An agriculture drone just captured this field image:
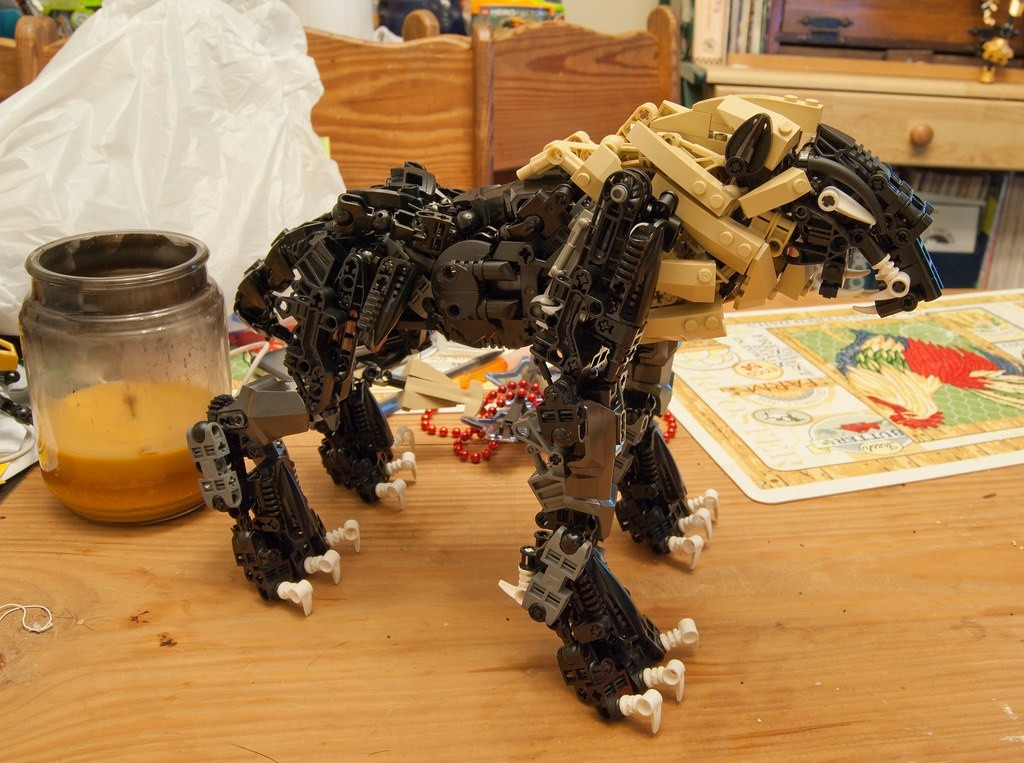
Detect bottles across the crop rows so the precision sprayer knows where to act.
[19,230,230,525]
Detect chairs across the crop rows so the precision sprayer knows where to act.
[402,3,683,179]
[0,14,61,103]
[225,22,493,191]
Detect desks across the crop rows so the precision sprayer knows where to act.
[699,54,1024,173]
[1,286,1024,762]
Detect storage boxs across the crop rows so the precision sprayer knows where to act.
[910,191,987,256]
